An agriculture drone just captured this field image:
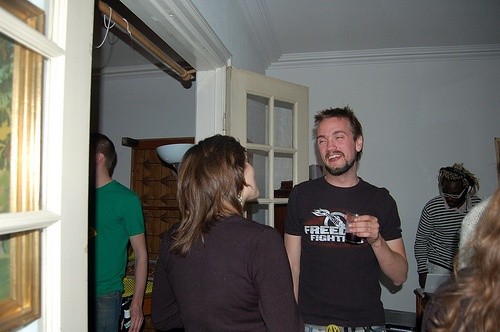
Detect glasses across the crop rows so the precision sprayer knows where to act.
[440,187,467,199]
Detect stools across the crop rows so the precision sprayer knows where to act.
[384,309,417,332]
[414,288,435,332]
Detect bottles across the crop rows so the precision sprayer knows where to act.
[122,307,132,332]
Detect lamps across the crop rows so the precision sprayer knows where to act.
[156,143,195,173]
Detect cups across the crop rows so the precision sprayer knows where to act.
[345,212,367,243]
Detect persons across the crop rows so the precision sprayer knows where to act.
[89,132,148,332]
[151,135,305,332]
[414,163,500,332]
[282,108,408,332]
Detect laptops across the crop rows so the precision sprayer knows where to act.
[421,274,451,293]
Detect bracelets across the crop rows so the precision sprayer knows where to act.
[369,234,380,243]
